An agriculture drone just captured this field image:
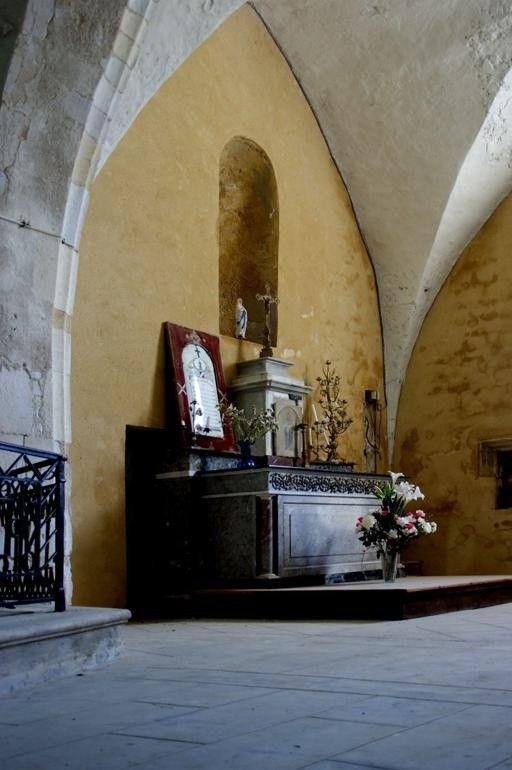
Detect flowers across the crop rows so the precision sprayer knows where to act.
[214,401,280,442]
[354,470,438,558]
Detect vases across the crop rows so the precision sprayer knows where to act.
[380,540,399,582]
[236,439,255,469]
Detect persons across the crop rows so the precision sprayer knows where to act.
[234,296,248,339]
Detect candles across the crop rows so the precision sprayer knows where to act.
[312,403,318,421]
[323,432,330,445]
[308,427,313,446]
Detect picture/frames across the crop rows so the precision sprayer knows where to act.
[164,321,235,452]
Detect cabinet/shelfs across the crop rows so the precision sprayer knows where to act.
[155,466,413,586]
